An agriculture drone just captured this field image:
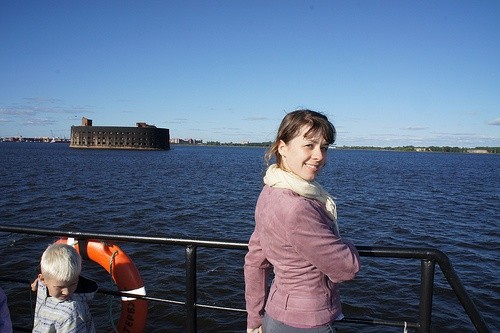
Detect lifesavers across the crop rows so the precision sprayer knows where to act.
[48,237,148,333]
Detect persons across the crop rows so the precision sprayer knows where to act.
[242,108,361,333]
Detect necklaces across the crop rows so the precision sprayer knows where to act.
[32,243,100,333]
